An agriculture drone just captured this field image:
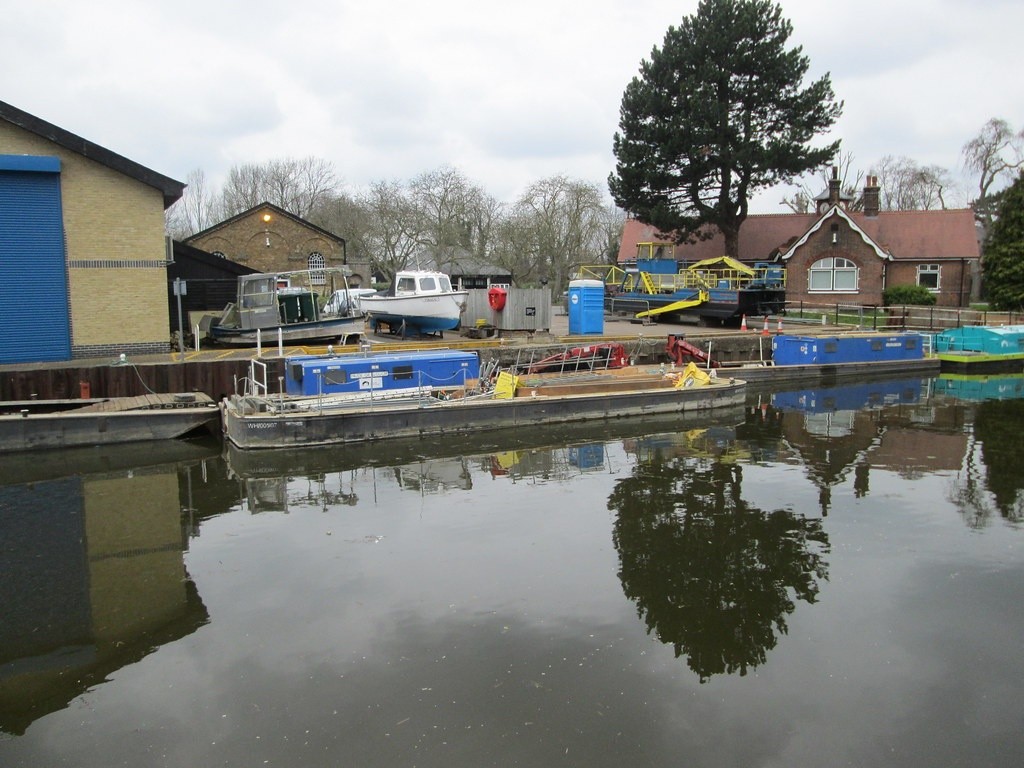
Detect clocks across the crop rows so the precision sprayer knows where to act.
[840,201,847,212]
[819,202,830,216]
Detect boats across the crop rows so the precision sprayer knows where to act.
[929,323,1024,371]
[529,327,941,385]
[1,388,216,451]
[206,267,366,346]
[357,269,470,337]
[219,334,748,448]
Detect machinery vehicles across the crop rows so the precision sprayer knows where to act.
[601,239,788,329]
